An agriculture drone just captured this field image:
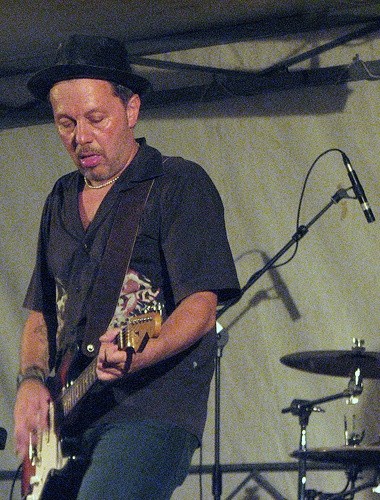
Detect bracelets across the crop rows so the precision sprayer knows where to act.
[17,365,46,388]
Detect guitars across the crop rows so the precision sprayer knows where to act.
[21,302,165,500]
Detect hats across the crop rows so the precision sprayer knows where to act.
[26,34,152,110]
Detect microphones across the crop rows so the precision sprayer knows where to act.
[341,151,376,225]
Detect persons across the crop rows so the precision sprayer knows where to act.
[12,33,243,500]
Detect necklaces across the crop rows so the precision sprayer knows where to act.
[83,176,120,190]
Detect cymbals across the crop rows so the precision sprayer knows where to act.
[288,446,380,464]
[281,351,380,378]
